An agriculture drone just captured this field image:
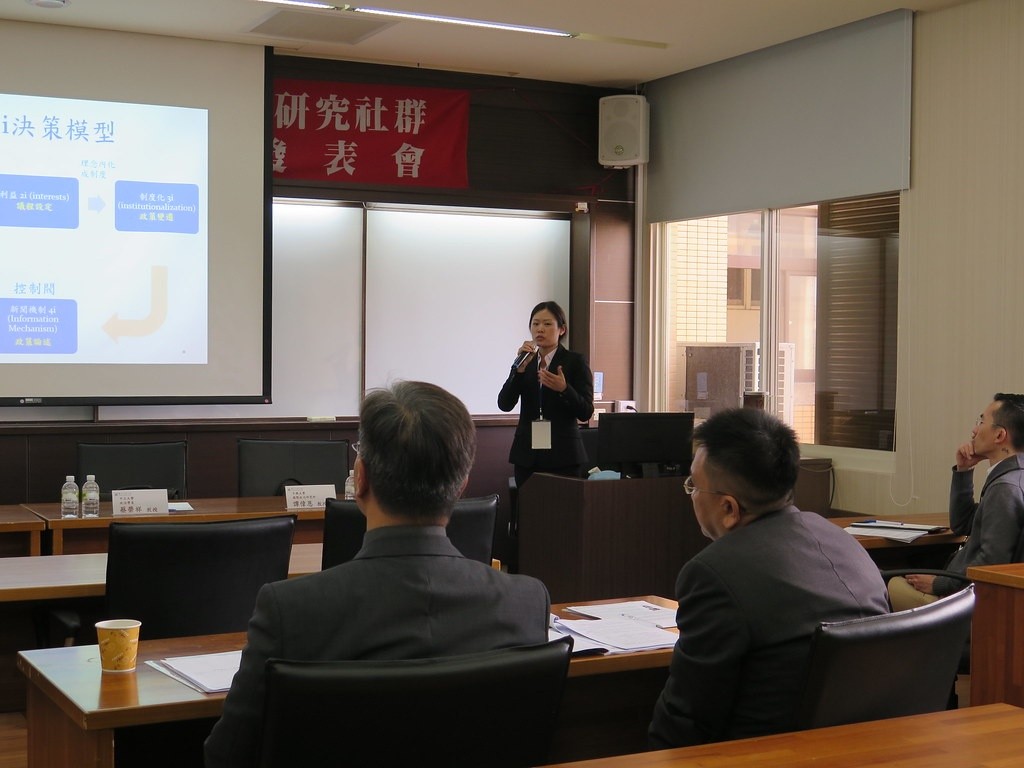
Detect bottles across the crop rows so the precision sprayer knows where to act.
[61,476,79,518]
[344,470,356,500]
[82,475,99,518]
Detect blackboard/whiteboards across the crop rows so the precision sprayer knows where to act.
[93,198,592,427]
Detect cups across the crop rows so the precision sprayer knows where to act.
[95,620,141,672]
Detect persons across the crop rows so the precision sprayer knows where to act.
[497,302,595,491]
[644,406,891,751]
[887,392,1024,614]
[201,379,549,768]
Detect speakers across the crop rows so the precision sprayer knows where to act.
[598,95,650,169]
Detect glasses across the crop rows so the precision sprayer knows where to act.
[682,475,742,511]
[351,441,361,457]
[976,417,1007,435]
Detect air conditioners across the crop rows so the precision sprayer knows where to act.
[684,343,795,431]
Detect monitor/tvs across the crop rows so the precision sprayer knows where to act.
[596,412,694,480]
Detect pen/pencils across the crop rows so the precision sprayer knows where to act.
[621,613,664,630]
[875,520,904,525]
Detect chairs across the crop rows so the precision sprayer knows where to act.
[237,437,349,498]
[797,581,977,729]
[74,441,189,500]
[322,492,500,573]
[36,510,300,648]
[261,637,575,767]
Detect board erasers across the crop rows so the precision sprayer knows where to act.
[307,416,336,422]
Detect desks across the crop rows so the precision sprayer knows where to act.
[1,492,1023,767]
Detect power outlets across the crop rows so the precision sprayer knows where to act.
[614,400,637,412]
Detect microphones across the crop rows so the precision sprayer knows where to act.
[512,339,537,370]
